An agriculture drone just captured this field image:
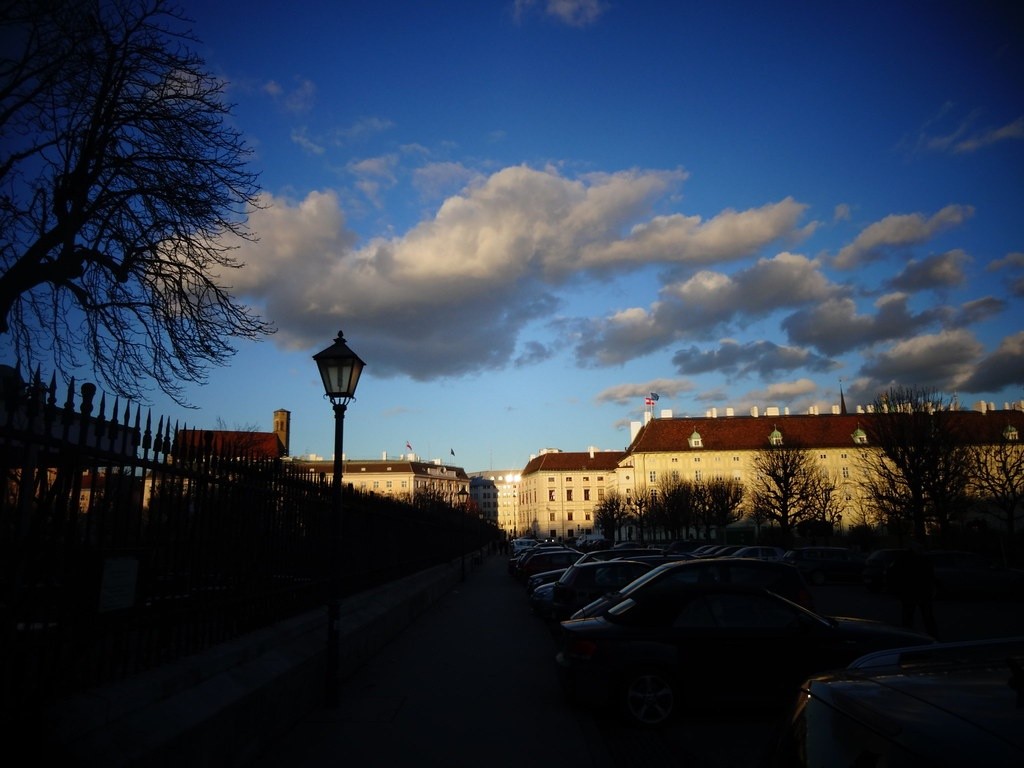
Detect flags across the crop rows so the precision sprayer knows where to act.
[645,397,655,406]
[407,441,412,451]
[451,448,455,456]
[650,392,660,401]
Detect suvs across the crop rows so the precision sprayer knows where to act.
[863,548,944,594]
[781,546,867,587]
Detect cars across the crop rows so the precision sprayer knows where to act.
[552,583,942,733]
[761,635,1024,768]
[690,543,786,558]
[561,557,818,621]
[508,536,694,622]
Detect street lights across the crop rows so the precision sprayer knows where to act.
[458,486,468,583]
[478,510,484,563]
[486,516,497,556]
[313,331,366,711]
